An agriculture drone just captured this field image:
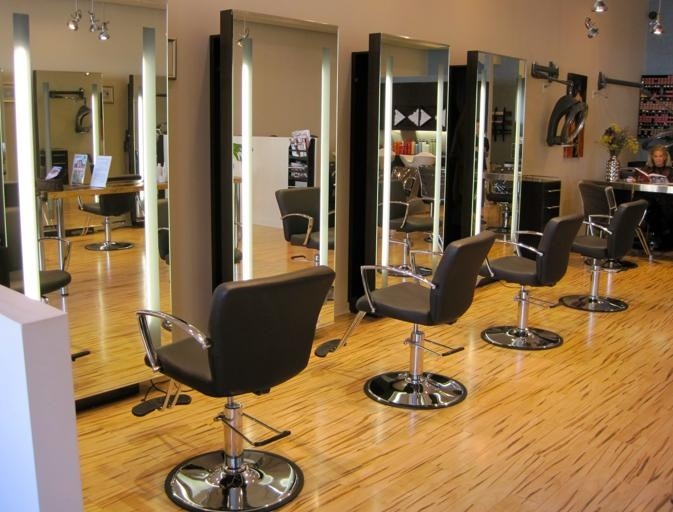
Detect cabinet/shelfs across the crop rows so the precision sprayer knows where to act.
[520,174,561,262]
[288,138,314,188]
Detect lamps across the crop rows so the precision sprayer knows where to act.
[582,16,599,39]
[646,0,665,35]
[590,0,608,12]
[66,0,110,41]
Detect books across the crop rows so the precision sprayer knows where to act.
[290,130,311,180]
[635,167,669,183]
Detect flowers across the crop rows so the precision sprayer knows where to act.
[593,121,637,157]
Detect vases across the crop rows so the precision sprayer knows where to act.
[603,155,620,182]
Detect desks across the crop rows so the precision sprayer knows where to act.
[43,182,168,298]
[577,177,672,261]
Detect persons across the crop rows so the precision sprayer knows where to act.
[626,144,673,183]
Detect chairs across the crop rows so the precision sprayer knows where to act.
[76,191,138,252]
[269,184,338,303]
[5,205,89,361]
[557,199,646,312]
[122,267,338,510]
[417,164,445,243]
[484,173,513,234]
[475,210,590,350]
[575,181,653,271]
[313,229,496,409]
[377,178,443,277]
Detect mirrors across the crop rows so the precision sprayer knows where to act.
[31,69,106,190]
[209,8,338,332]
[132,76,166,223]
[0,0,173,409]
[468,51,527,285]
[357,32,449,319]
